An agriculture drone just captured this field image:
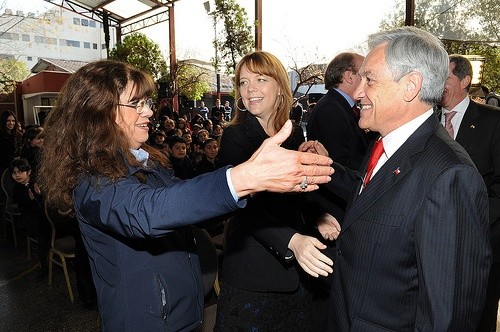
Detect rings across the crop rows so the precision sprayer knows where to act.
[300,176,307,189]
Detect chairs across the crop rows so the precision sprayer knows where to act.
[44,195,77,301]
[1,167,21,246]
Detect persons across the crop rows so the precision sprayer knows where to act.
[435,54,500,332]
[309,52,375,178]
[41,60,335,332]
[290,98,303,126]
[1,98,234,308]
[214,52,342,332]
[303,99,317,118]
[479,85,500,107]
[331,25,490,332]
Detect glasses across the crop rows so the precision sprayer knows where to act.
[118,99,152,115]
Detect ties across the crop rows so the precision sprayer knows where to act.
[363,138,384,189]
[443,111,457,140]
